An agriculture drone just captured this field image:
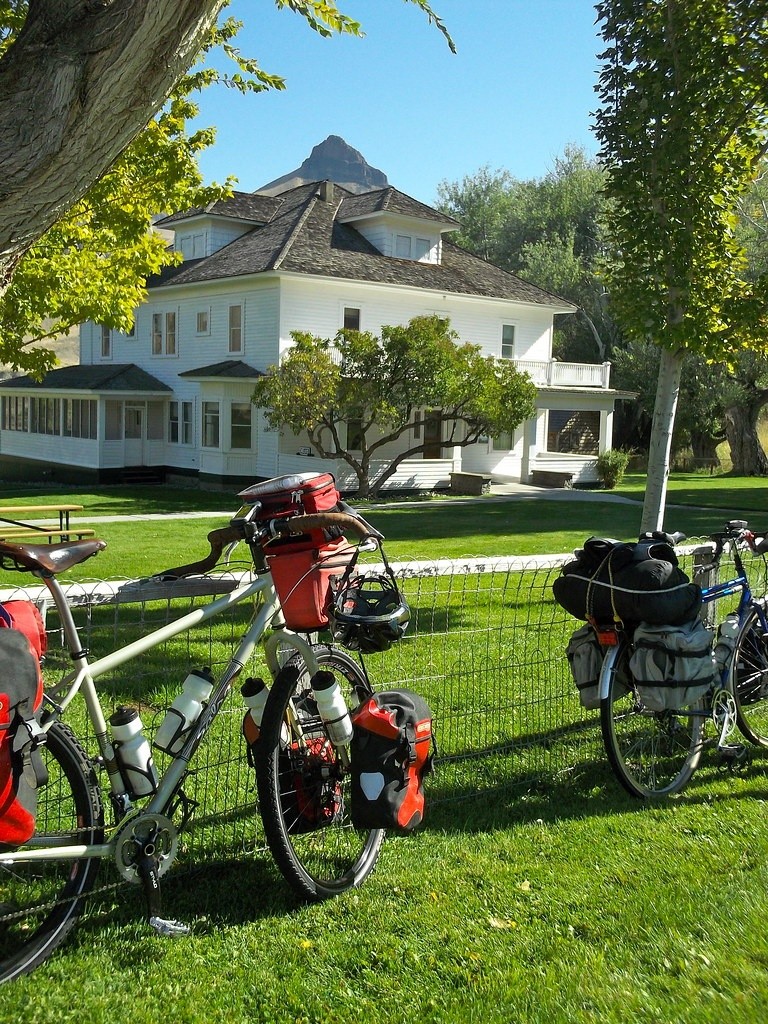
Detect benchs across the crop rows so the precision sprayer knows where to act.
[0,528,96,552]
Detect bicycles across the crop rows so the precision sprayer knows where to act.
[1,495,386,984]
[596,518,766,798]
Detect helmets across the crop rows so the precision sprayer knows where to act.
[324,573,412,655]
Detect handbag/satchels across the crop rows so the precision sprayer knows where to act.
[242,708,343,835]
[236,471,348,555]
[0,599,47,854]
[349,688,438,831]
[629,614,716,713]
[266,535,359,632]
[564,623,636,710]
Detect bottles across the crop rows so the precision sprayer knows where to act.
[308,671,355,744]
[713,611,740,670]
[108,706,158,796]
[154,663,215,755]
[241,677,292,751]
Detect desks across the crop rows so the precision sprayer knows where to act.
[0,503,84,544]
